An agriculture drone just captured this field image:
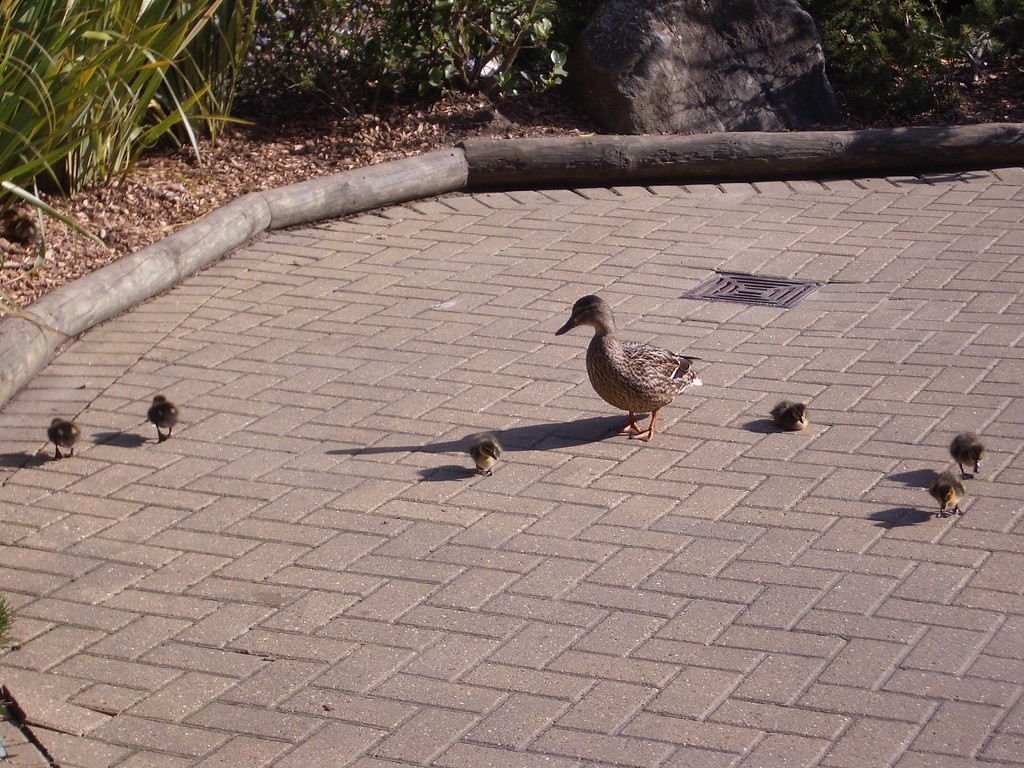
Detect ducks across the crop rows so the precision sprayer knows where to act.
[47,417,81,461]
[768,400,809,432]
[929,470,966,518]
[470,433,501,477]
[949,432,985,481]
[555,295,705,443]
[146,394,179,443]
